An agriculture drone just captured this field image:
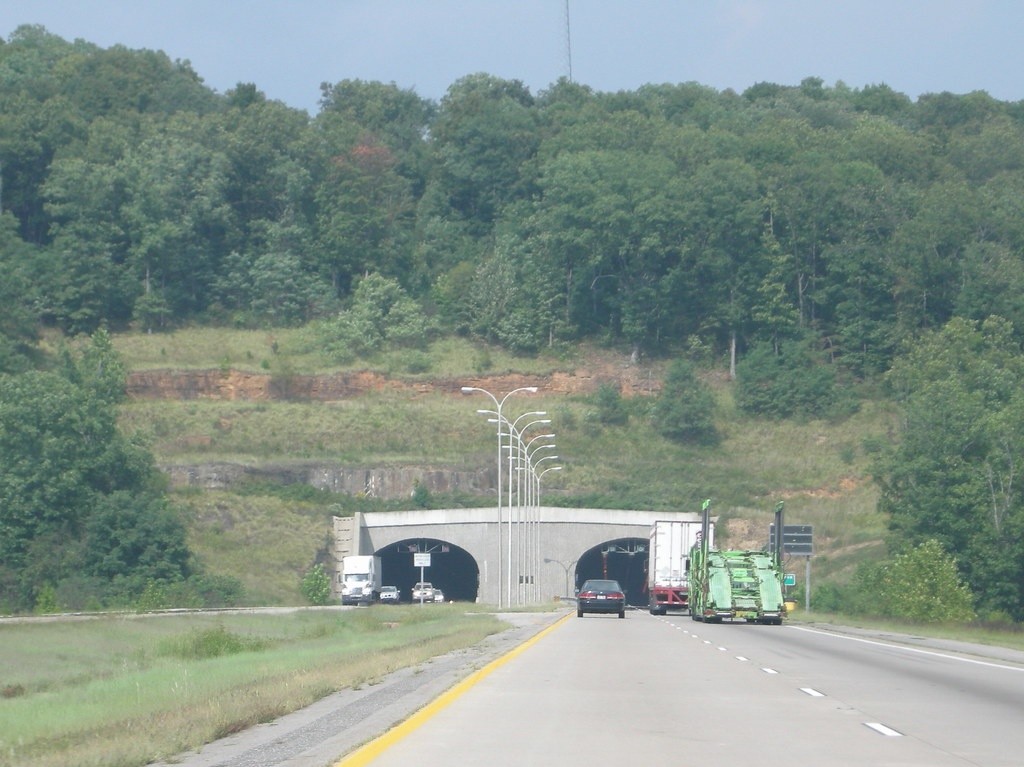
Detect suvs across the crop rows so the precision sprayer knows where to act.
[411,581,436,603]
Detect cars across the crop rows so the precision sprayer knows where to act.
[379,585,402,605]
[573,579,628,619]
[434,589,445,602]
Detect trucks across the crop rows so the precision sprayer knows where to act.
[643,520,716,616]
[337,556,383,606]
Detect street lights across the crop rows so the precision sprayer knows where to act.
[515,465,563,601]
[496,432,556,606]
[476,409,546,608]
[506,455,560,603]
[487,419,551,606]
[462,386,538,610]
[503,444,555,604]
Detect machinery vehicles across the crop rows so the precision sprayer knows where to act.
[683,497,786,625]
